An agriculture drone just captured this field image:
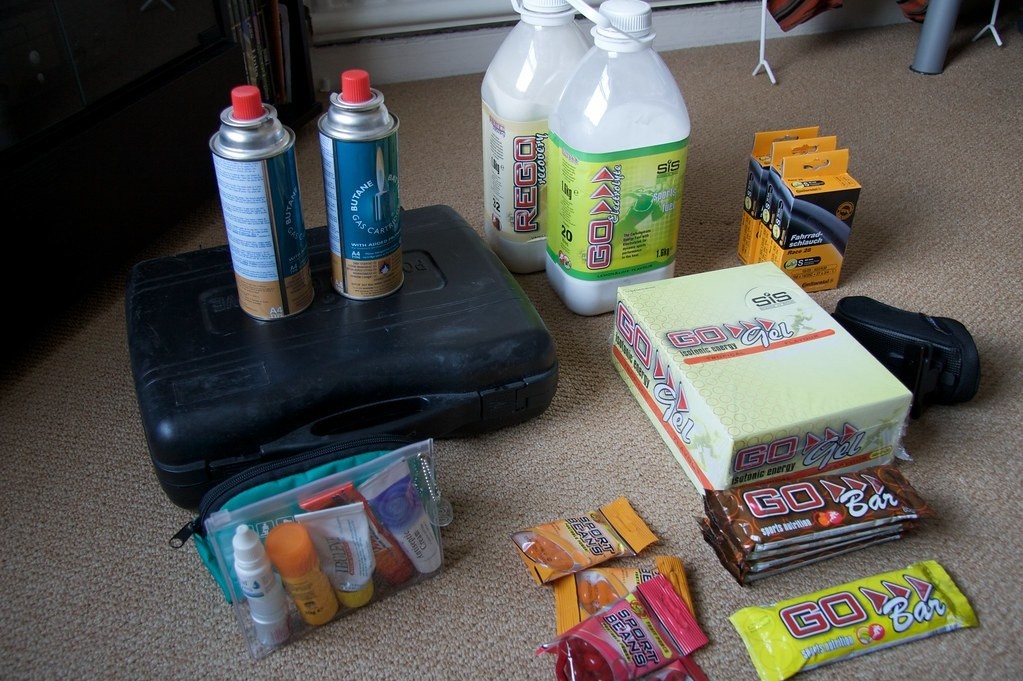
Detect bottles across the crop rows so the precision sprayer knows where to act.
[317,69,404,301]
[231,524,275,599]
[248,572,292,646]
[209,85,315,321]
[265,521,338,626]
[546,0,690,316]
[481,0,594,275]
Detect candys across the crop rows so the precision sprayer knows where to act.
[510,462,981,681]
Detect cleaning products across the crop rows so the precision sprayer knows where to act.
[480,0,692,318]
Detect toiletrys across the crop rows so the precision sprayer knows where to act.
[204,438,454,663]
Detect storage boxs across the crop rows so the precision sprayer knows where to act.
[123,201,559,512]
[736,126,862,288]
[611,259,913,498]
